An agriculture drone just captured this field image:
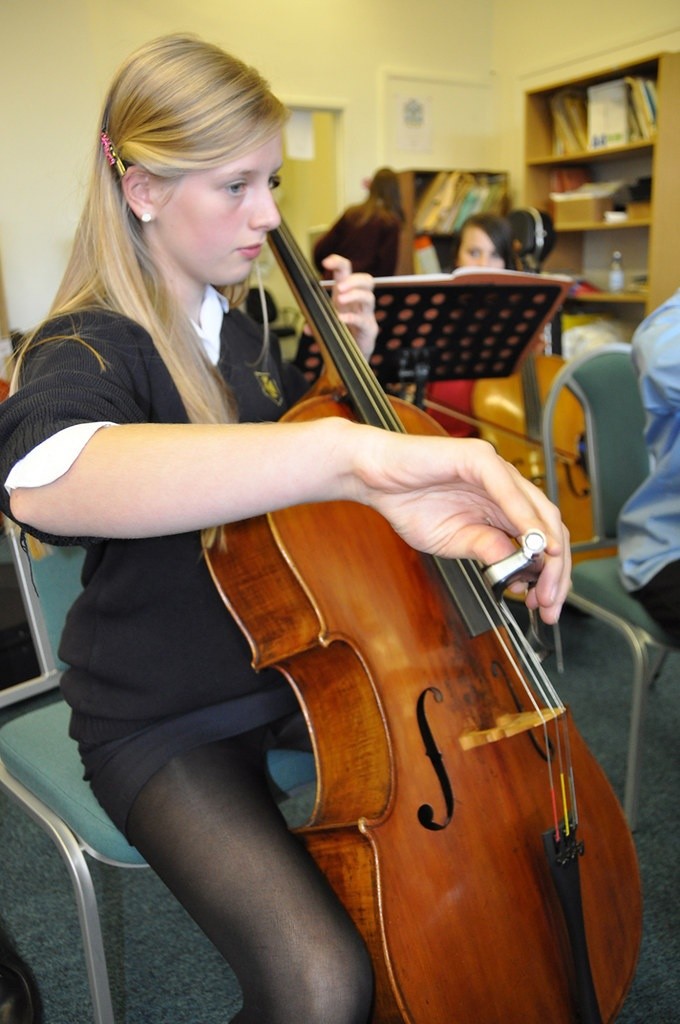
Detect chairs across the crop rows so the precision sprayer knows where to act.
[247,287,297,337]
[1,517,317,1023]
[544,350,680,834]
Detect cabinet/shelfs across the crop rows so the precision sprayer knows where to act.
[393,170,511,276]
[524,51,680,318]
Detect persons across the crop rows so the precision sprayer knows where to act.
[1,35,571,1023]
[617,289,680,644]
[311,167,513,278]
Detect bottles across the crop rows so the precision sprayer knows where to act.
[609,251,626,295]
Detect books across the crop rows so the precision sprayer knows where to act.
[547,73,658,155]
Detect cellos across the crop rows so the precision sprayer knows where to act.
[201,201,644,1023]
[470,237,617,601]
